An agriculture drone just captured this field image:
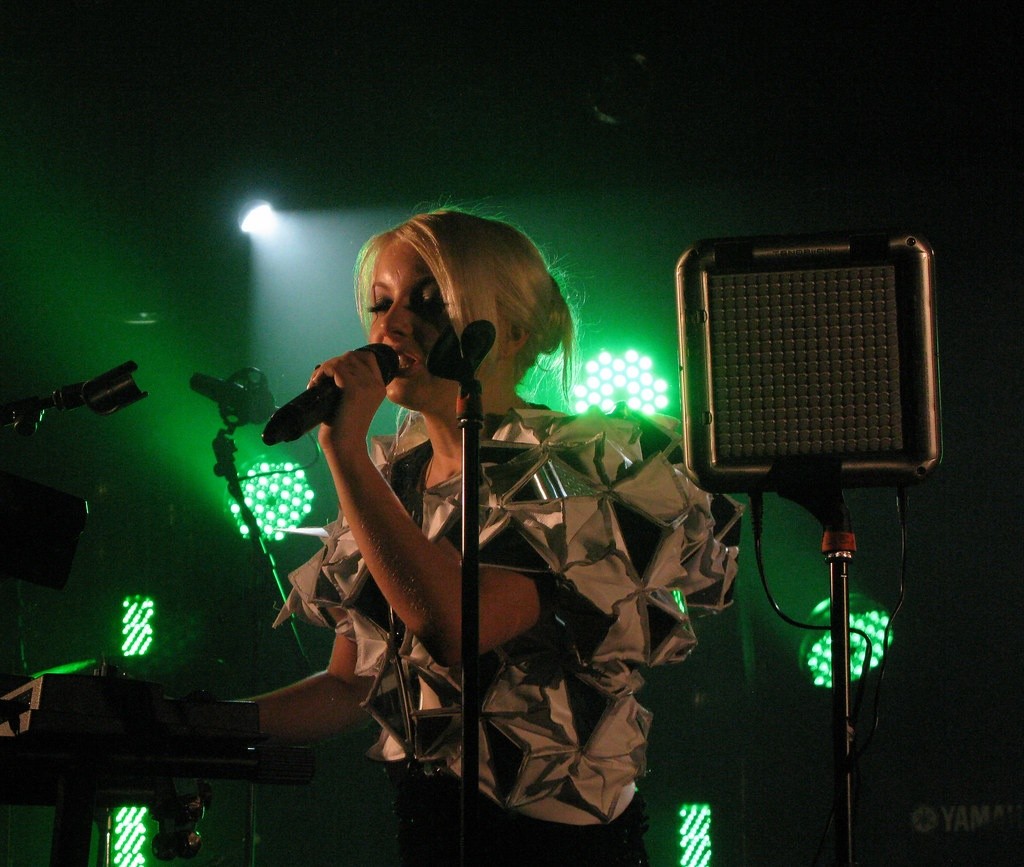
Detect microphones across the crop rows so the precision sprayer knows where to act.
[261,343,399,447]
[186,373,283,422]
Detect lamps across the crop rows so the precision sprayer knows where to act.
[798,586,896,689]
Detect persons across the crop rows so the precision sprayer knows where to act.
[134,206,735,867]
